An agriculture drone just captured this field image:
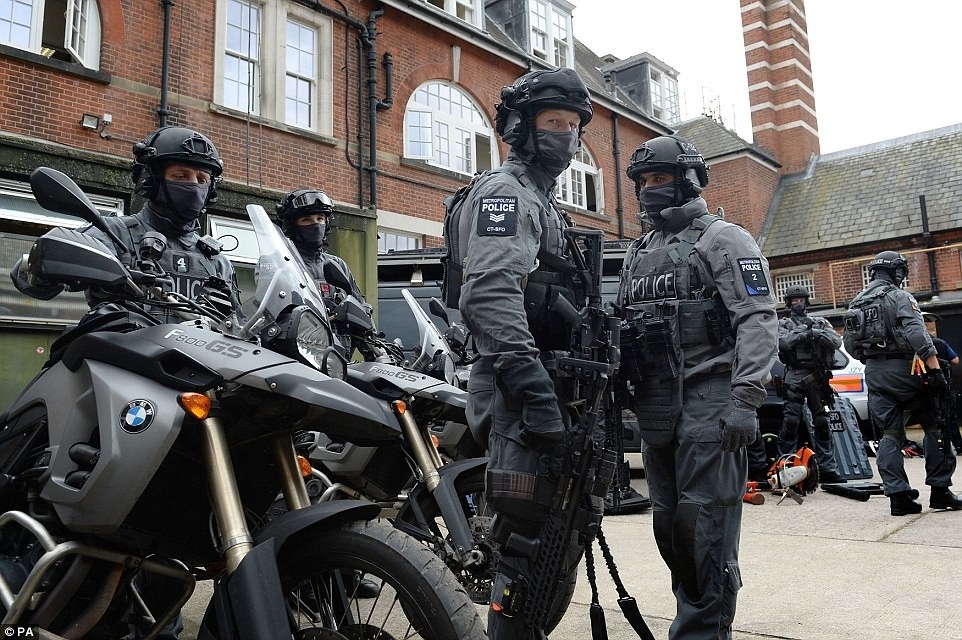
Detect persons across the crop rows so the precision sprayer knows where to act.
[779,285,845,481]
[10,128,243,320]
[453,67,595,640]
[273,188,372,362]
[846,252,962,515]
[615,137,778,640]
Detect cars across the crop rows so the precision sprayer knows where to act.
[757,336,874,441]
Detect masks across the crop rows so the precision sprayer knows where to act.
[295,223,326,248]
[524,126,578,163]
[638,181,684,216]
[157,179,210,217]
[793,303,804,312]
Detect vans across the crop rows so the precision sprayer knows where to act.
[377,239,644,454]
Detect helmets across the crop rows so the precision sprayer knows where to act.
[280,191,331,221]
[627,137,710,187]
[502,67,593,148]
[785,286,808,296]
[868,251,909,278]
[135,126,222,196]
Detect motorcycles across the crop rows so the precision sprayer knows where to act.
[0,165,496,640]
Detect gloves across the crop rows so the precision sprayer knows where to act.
[929,371,945,399]
[718,398,758,453]
[522,400,566,440]
[797,330,813,343]
[803,318,815,328]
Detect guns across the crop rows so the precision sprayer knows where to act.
[797,341,838,412]
[485,357,611,640]
[770,374,784,398]
[922,359,962,456]
[547,227,622,425]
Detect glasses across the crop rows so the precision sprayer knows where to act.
[292,192,332,208]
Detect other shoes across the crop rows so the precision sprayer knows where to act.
[818,471,847,485]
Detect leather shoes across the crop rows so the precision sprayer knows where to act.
[929,486,962,508]
[891,491,922,516]
